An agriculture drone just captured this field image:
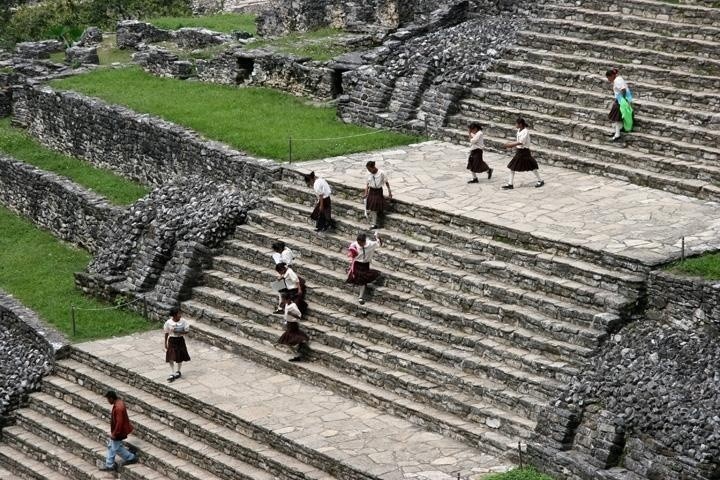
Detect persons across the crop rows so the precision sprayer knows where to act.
[276,295,309,362]
[606,69,634,142]
[161,308,193,381]
[466,121,494,184]
[99,390,137,472]
[303,170,338,232]
[274,262,309,312]
[501,117,545,190]
[269,240,295,270]
[342,232,383,304]
[359,159,393,229]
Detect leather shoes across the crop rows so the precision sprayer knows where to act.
[288,357,301,361]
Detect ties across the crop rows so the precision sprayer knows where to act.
[362,247,366,260]
[373,176,378,188]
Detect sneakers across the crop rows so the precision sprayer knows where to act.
[467,177,480,183]
[534,179,545,187]
[612,135,621,142]
[99,456,137,472]
[487,168,493,179]
[358,297,364,305]
[166,375,175,381]
[500,183,514,190]
[174,371,182,379]
